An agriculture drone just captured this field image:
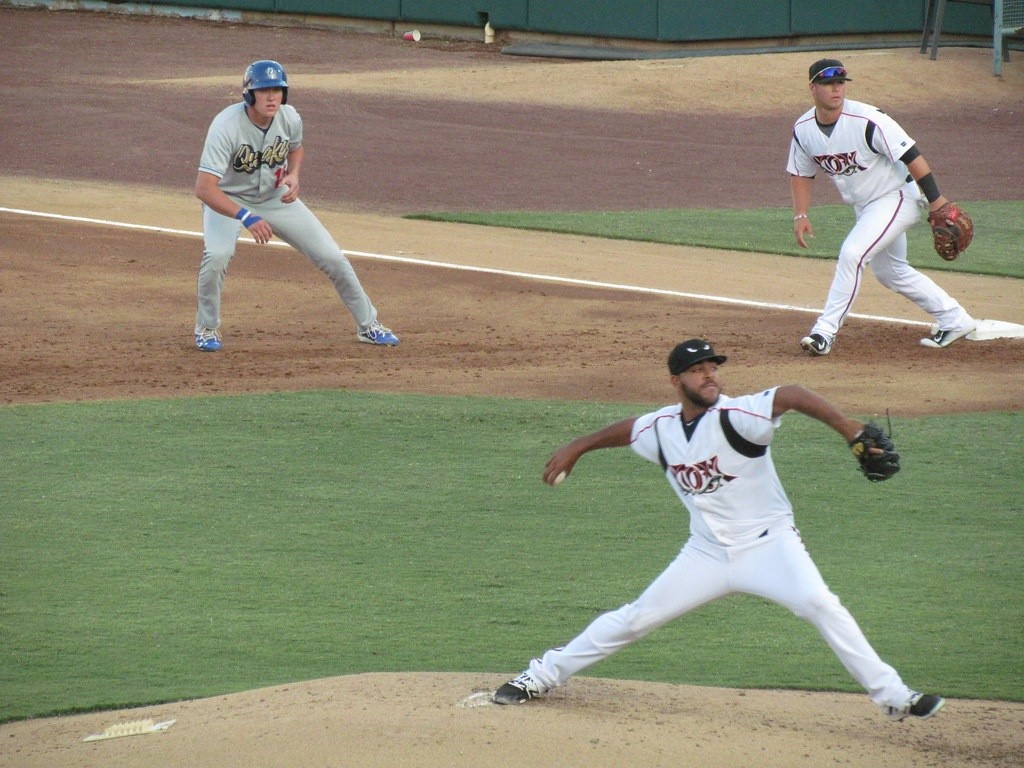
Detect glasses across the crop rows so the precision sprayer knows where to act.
[808,66,847,85]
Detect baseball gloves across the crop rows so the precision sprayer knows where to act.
[927,201,975,261]
[847,420,901,483]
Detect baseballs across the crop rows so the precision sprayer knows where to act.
[548,470,566,486]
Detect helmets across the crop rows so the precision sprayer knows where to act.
[241,60,289,106]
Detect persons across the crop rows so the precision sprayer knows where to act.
[194,59,399,351]
[787,59,977,355]
[491,339,947,723]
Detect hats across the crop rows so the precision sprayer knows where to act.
[808,58,852,84]
[668,339,727,376]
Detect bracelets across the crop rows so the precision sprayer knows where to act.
[240,211,252,224]
[794,214,807,220]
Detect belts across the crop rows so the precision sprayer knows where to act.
[760,529,768,538]
[905,174,914,183]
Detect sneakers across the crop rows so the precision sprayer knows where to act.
[493,672,540,705]
[883,689,946,722]
[194,322,222,352]
[800,333,831,355]
[920,313,977,348]
[357,320,400,347]
